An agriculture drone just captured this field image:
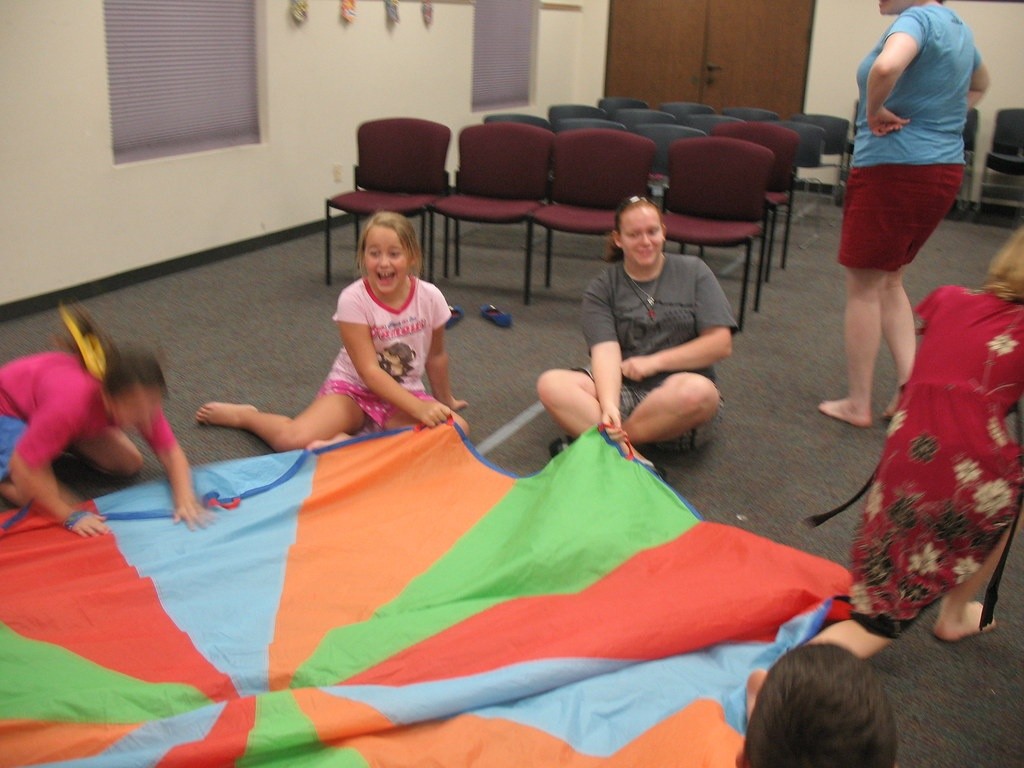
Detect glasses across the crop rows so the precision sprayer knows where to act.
[623,194,659,208]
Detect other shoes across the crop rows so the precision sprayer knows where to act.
[653,429,696,452]
[547,435,573,459]
[445,305,464,330]
[479,304,512,326]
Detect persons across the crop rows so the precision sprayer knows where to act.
[737,643,899,768]
[819,0,991,426]
[536,195,739,474]
[746,226,1024,721]
[194,211,470,459]
[0,311,219,537]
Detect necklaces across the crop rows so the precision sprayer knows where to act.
[622,253,668,319]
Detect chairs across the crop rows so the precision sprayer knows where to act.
[324,101,1024,332]
